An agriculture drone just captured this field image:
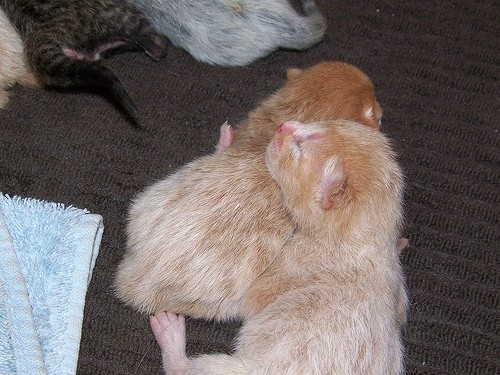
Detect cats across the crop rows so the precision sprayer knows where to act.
[0,0,328,131]
[111,61,411,375]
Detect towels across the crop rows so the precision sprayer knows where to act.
[0,192,104,375]
